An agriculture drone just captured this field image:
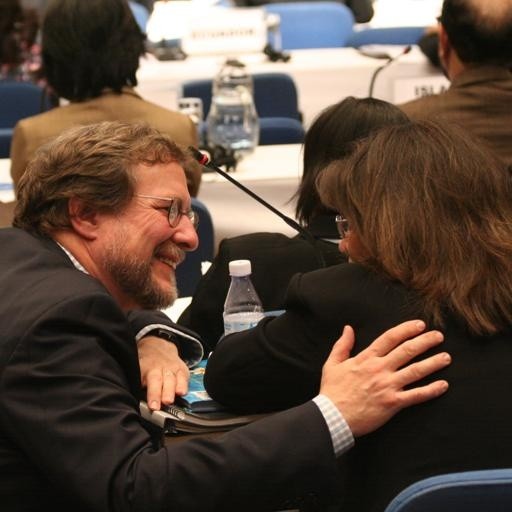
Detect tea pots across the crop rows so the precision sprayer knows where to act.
[204,56,258,161]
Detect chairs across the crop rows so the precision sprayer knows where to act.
[0,1,511,512]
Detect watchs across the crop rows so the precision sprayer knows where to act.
[144,326,183,345]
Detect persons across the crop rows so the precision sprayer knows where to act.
[176,97,415,354]
[1,120,451,511]
[10,0,203,198]
[418,23,447,78]
[0,1,59,157]
[395,0,511,162]
[205,121,510,510]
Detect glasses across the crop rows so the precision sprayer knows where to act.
[335,214,349,241]
[93,193,199,229]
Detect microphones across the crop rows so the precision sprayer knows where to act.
[368,44,413,98]
[187,145,329,270]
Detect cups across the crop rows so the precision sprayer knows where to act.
[176,97,202,128]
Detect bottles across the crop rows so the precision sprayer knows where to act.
[222,260,263,330]
